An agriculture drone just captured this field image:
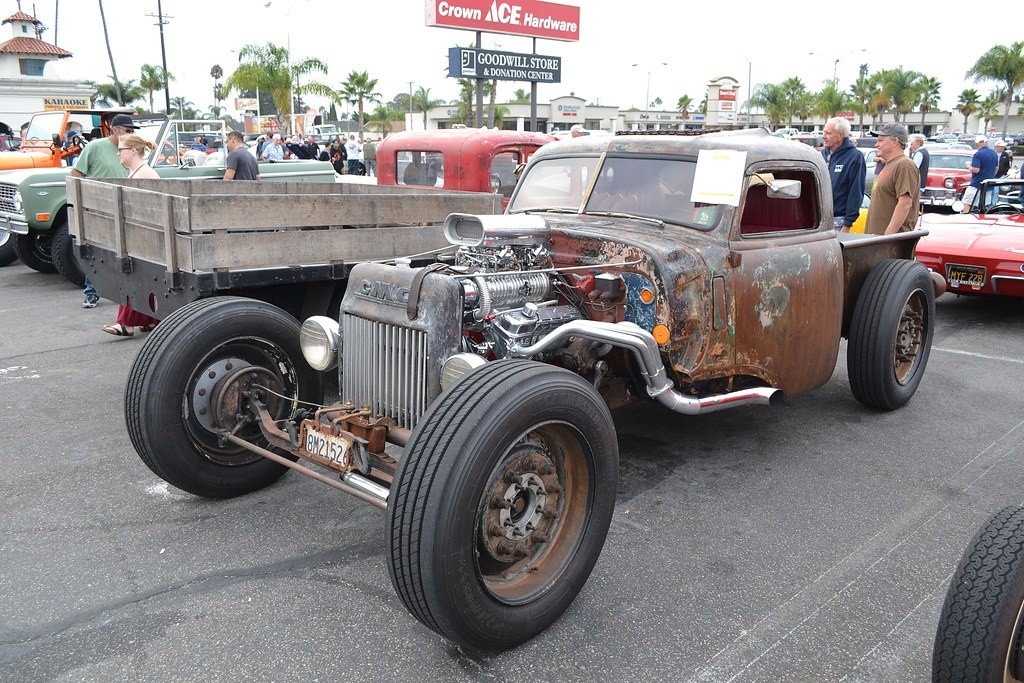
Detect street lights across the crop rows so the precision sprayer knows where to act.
[632,62,668,130]
[807,48,867,90]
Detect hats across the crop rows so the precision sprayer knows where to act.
[210,140,222,148]
[571,125,590,136]
[870,122,908,147]
[994,140,1008,147]
[111,114,140,129]
[974,135,988,143]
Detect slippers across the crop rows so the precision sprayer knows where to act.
[102,322,134,336]
[140,324,158,332]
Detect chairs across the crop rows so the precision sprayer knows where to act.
[181,150,207,166]
[203,151,225,167]
[403,162,438,187]
[741,183,813,234]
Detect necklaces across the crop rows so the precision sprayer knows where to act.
[130,163,147,177]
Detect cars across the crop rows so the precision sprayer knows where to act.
[772,127,1024,158]
[848,192,871,235]
[856,147,879,195]
[914,177,1024,301]
[919,150,976,214]
[0,135,21,151]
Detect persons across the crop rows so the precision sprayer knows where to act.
[570,125,583,138]
[59,114,378,336]
[863,124,921,236]
[849,133,1024,214]
[818,116,866,233]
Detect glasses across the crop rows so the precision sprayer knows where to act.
[118,148,139,152]
[226,137,235,144]
[115,126,135,135]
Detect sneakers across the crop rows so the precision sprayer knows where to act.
[82,294,99,308]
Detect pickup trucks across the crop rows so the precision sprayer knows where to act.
[0,120,336,287]
[0,107,185,172]
[124,131,948,653]
[62,131,557,316]
